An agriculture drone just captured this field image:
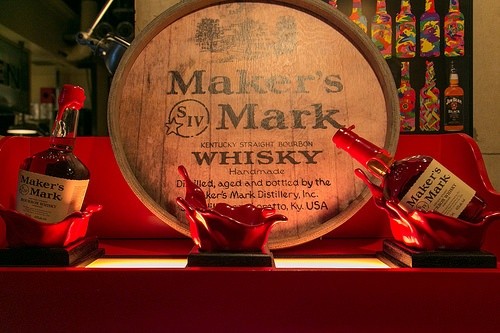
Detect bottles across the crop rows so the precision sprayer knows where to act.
[332,128,487,222]
[16,85,90,223]
